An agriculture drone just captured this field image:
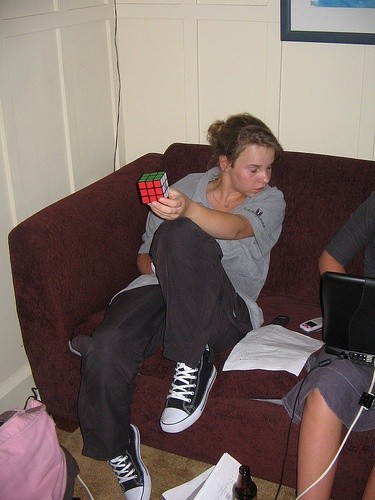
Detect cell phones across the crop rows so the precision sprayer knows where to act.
[300,317,322,332]
[270,316,290,327]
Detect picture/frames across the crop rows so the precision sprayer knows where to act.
[280,0,375,46]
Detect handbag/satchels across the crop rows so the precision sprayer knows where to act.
[0,387,67,500]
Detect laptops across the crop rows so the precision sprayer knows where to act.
[318,270,375,362]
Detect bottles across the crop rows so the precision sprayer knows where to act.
[231,465,258,500]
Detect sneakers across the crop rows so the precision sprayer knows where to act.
[107,423,152,500]
[160,345,218,433]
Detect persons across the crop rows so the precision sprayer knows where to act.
[79,111,287,500]
[282,190,375,500]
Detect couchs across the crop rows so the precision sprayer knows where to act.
[8,143,375,500]
